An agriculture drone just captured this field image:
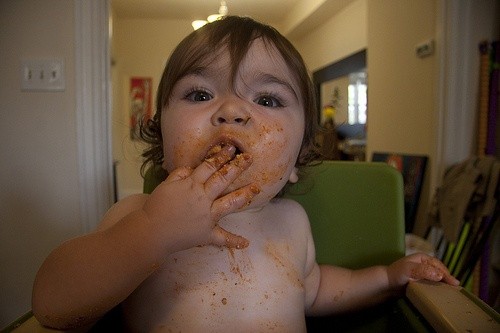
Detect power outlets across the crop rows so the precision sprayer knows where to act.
[416,40,434,59]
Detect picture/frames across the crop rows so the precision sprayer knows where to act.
[370,153,429,234]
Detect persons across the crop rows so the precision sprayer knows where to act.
[32,15,463,333]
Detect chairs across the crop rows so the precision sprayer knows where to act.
[142,161,429,333]
